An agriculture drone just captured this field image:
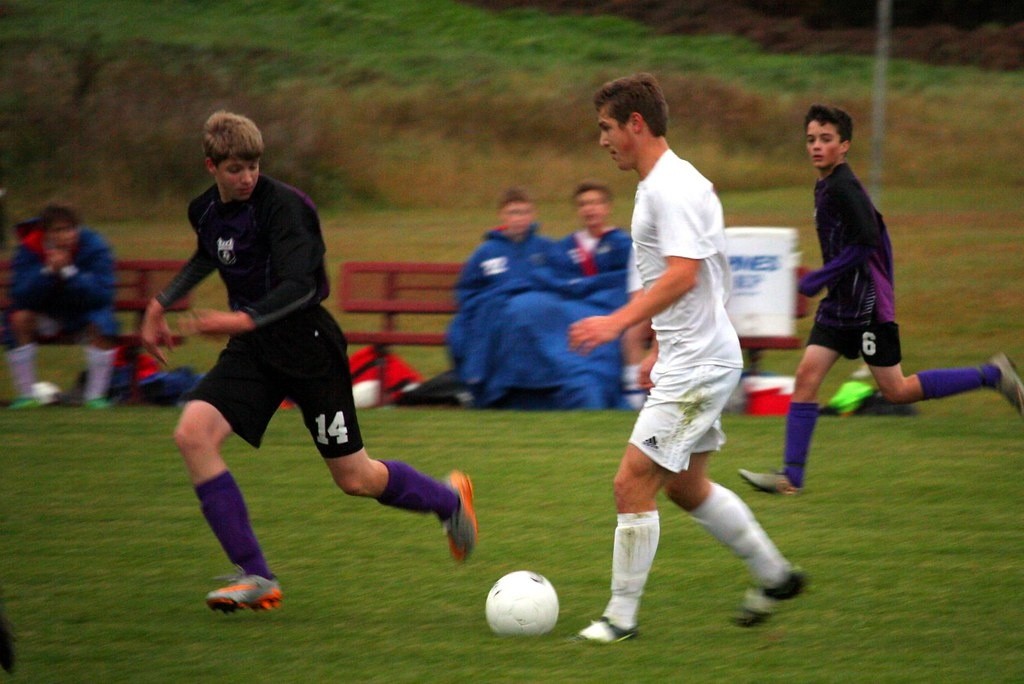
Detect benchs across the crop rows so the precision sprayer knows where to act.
[337,260,814,404]
[0,259,188,407]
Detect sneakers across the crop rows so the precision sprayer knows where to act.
[731,566,808,629]
[575,615,639,646]
[436,471,480,562]
[205,575,283,611]
[739,467,804,496]
[985,353,1024,415]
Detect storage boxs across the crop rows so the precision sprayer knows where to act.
[740,377,795,417]
[721,226,798,337]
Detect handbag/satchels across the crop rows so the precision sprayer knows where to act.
[77,351,197,405]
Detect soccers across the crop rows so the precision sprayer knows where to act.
[484,569,560,638]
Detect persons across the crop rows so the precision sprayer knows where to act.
[565,69,810,643]
[2,203,124,411]
[737,104,1024,497]
[452,182,648,411]
[142,111,479,613]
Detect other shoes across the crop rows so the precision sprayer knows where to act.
[82,398,110,411]
[11,397,43,410]
